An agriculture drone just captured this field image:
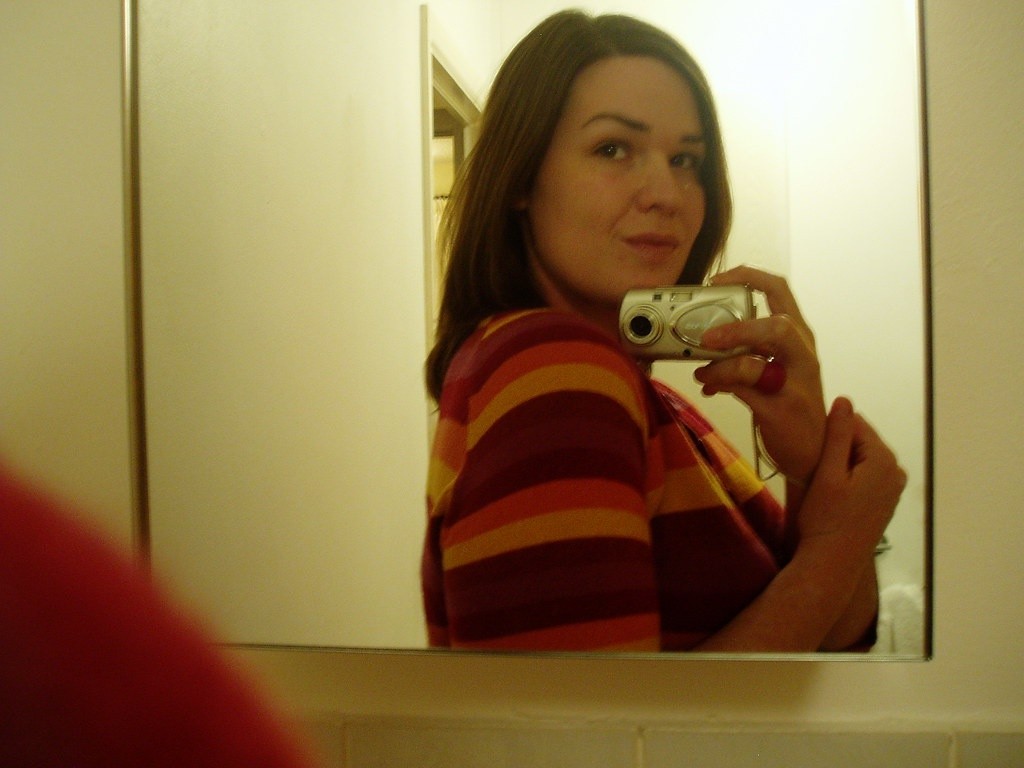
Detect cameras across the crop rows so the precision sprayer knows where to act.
[619,284,759,360]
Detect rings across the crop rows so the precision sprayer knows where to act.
[753,360,786,396]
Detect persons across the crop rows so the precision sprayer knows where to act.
[421,7,907,653]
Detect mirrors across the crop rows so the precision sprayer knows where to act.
[120,0,935,665]
